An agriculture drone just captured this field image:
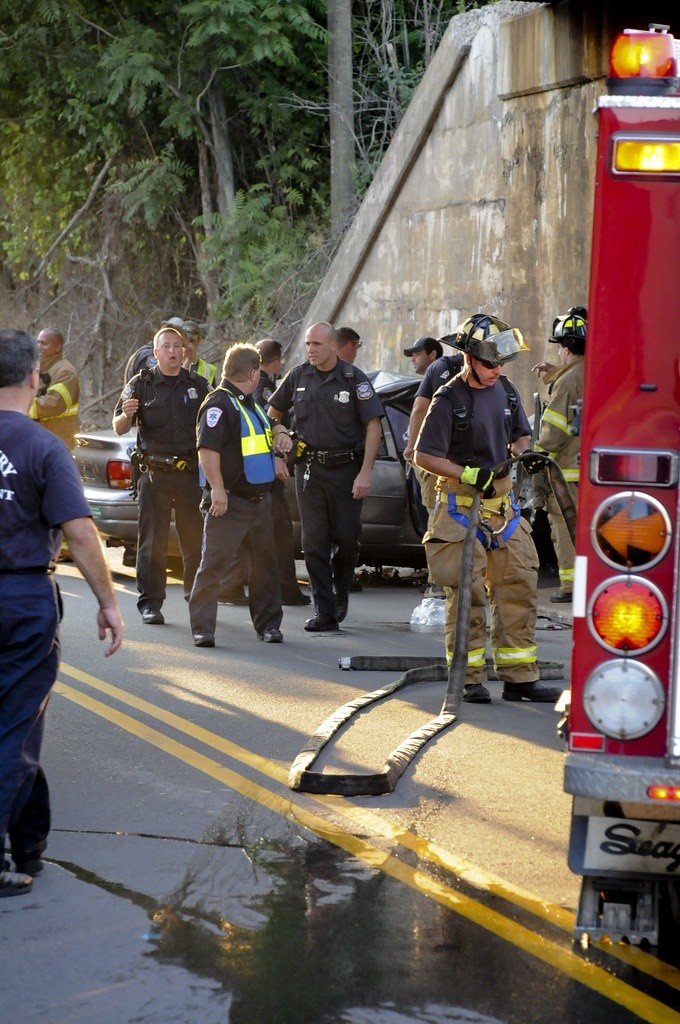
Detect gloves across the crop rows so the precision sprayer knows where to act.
[459,465,495,492]
[520,449,551,474]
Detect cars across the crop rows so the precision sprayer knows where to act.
[73,380,428,568]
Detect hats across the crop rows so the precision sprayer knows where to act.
[161,317,203,343]
[404,337,443,359]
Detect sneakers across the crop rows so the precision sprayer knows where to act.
[0,868,33,896]
[304,611,339,631]
[336,593,348,622]
[257,627,283,643]
[193,631,215,647]
[15,859,43,876]
[219,587,249,606]
[550,591,572,603]
[462,683,491,703]
[501,680,562,702]
[281,586,312,605]
[142,607,164,624]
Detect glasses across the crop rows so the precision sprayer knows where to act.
[469,354,505,368]
[268,358,285,364]
[352,341,362,347]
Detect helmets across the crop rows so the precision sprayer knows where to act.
[438,313,518,363]
[548,313,586,344]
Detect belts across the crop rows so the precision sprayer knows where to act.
[205,486,265,504]
[0,566,54,575]
[302,449,360,465]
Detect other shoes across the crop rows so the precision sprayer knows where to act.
[123,549,137,565]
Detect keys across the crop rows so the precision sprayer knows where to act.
[302,474,310,491]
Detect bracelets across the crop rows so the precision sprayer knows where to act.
[275,453,284,458]
[278,431,288,434]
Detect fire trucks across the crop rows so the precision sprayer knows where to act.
[552,30,680,945]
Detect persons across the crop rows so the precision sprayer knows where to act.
[335,327,360,365]
[253,339,281,404]
[191,343,293,648]
[1,329,124,896]
[29,329,80,558]
[113,327,211,622]
[405,314,563,704]
[403,337,443,374]
[531,306,585,601]
[125,316,218,386]
[267,321,385,632]
